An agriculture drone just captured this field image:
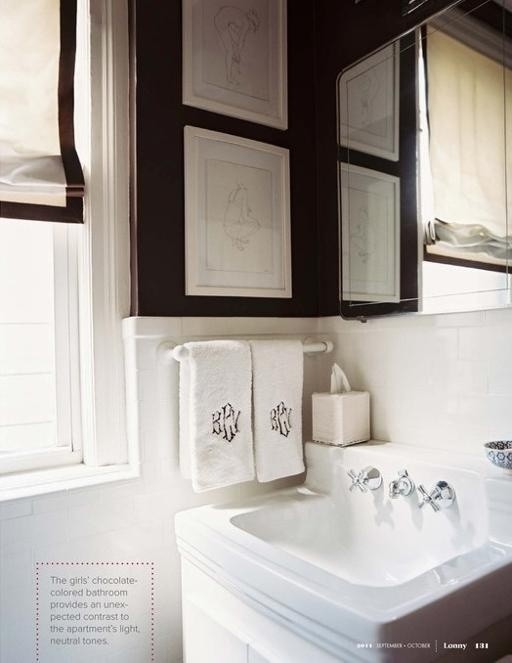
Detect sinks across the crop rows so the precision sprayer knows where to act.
[174,482,512,663]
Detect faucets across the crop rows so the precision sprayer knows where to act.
[389,477,413,498]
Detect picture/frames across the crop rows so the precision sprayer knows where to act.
[337,39,401,303]
[182,0,294,299]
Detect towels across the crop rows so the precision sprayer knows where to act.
[177,338,306,493]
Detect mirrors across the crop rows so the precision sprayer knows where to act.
[335,1,512,320]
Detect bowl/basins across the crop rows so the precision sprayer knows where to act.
[481,441,512,469]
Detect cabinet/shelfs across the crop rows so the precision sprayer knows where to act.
[180,555,341,663]
